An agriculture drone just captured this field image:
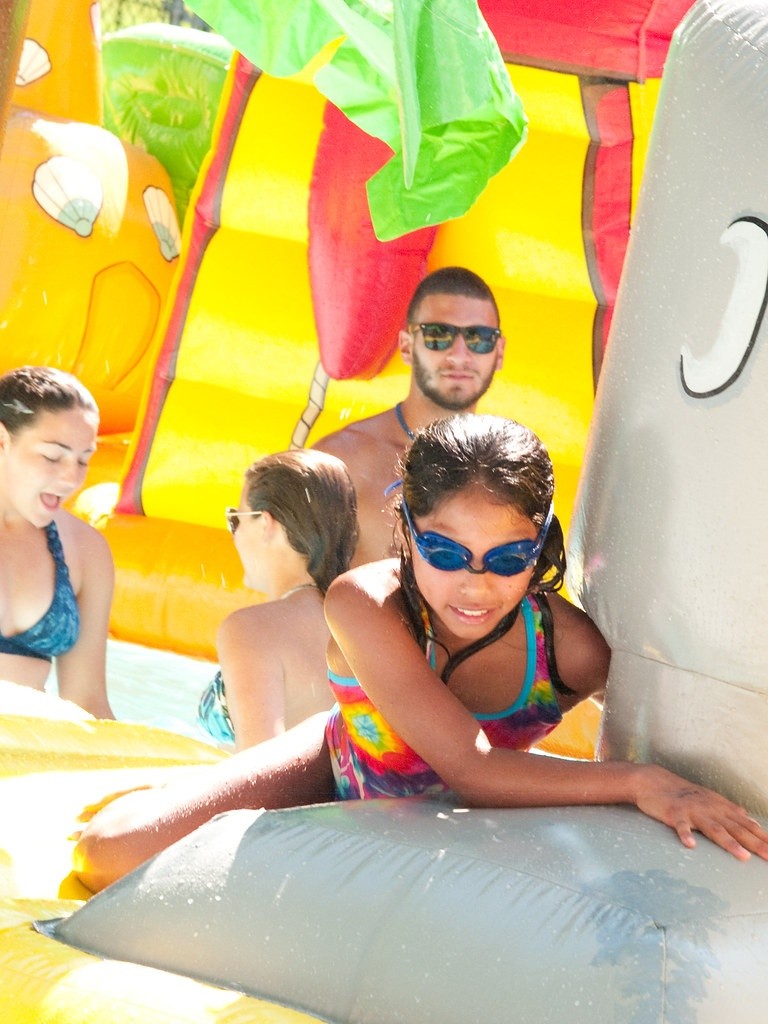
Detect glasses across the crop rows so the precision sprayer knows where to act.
[408,321,502,355]
[400,492,556,577]
[225,506,263,532]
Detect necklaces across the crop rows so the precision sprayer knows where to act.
[279,581,320,597]
[396,400,415,441]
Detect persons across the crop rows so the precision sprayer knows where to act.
[0,365,116,723]
[305,265,506,571]
[195,447,362,752]
[70,417,766,893]
